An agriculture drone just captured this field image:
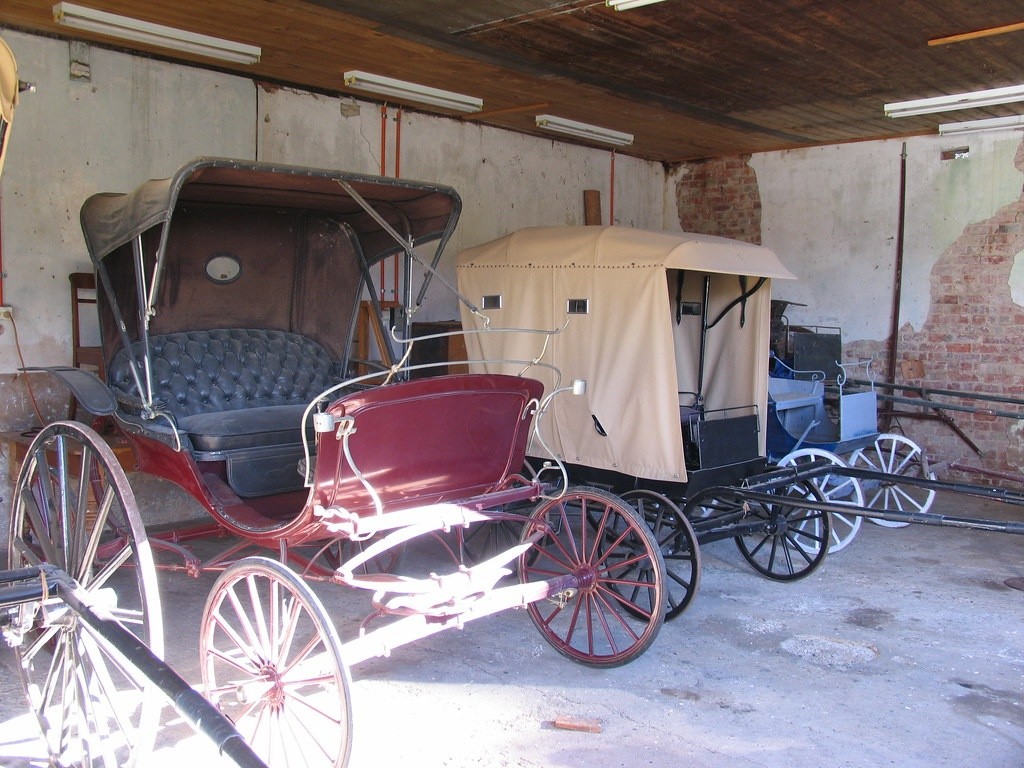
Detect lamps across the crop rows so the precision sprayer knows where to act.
[535,115,634,148]
[343,70,483,116]
[938,114,1024,136]
[53,2,262,67]
[883,83,1024,117]
[606,0,667,12]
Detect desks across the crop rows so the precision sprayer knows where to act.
[0,429,140,548]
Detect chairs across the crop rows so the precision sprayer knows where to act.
[67,272,105,420]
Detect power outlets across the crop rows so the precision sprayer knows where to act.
[0,307,13,321]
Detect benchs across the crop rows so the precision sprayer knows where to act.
[770,391,819,411]
[106,325,344,451]
[297,373,545,482]
[793,332,859,403]
